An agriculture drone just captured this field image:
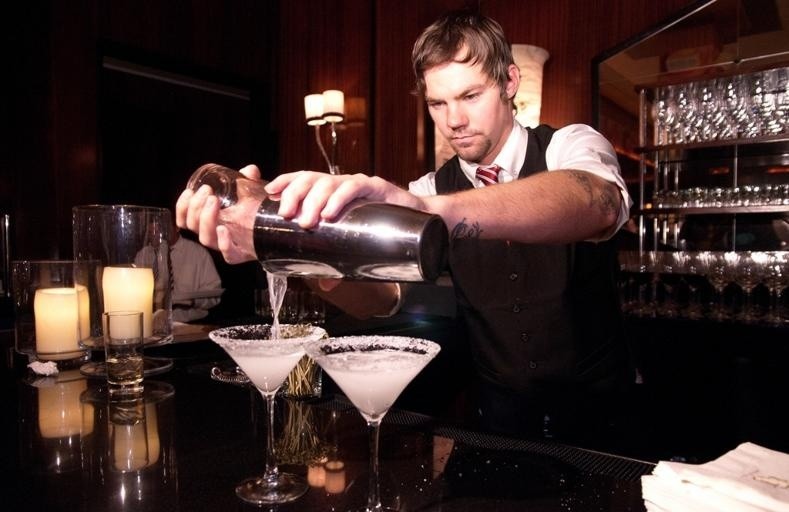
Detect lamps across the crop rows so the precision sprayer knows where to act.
[345,97,366,150]
[308,411,346,492]
[303,90,344,176]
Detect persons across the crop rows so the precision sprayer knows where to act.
[173,14,632,449]
[131,208,223,326]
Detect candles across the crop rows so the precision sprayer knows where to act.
[33,284,91,361]
[101,266,155,344]
[37,370,95,438]
[109,404,160,472]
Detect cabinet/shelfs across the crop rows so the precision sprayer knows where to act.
[638,51,789,464]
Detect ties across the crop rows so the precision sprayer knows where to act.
[153,249,174,292]
[476,164,500,185]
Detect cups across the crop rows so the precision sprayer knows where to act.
[206,322,328,506]
[621,249,788,299]
[71,205,174,381]
[653,184,789,209]
[304,333,443,510]
[183,164,269,261]
[101,310,144,407]
[654,67,789,144]
[253,190,450,286]
[6,259,102,372]
